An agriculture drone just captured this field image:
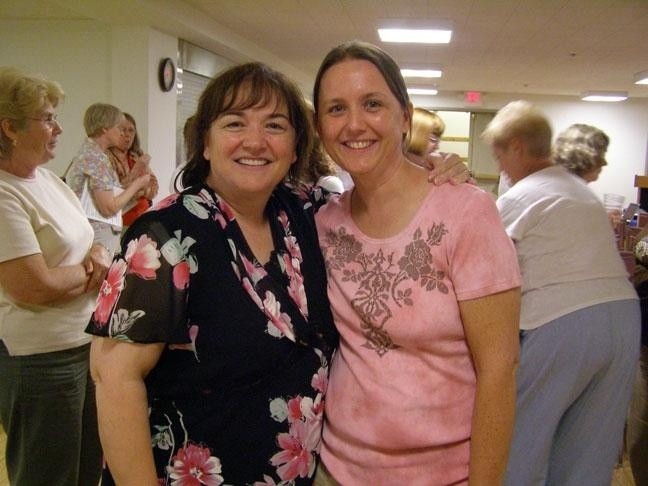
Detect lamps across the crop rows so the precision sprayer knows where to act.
[405,89,440,96]
[633,70,648,88]
[578,92,628,103]
[400,69,443,79]
[376,28,450,48]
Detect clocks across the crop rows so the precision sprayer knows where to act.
[161,58,177,93]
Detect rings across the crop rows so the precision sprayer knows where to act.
[464,169,474,178]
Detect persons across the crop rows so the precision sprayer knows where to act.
[296,99,344,194]
[110,113,160,237]
[314,40,522,486]
[400,104,444,161]
[65,102,150,256]
[479,98,640,485]
[0,66,115,485]
[551,121,610,184]
[85,63,471,486]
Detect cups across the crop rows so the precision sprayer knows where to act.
[604,194,625,218]
